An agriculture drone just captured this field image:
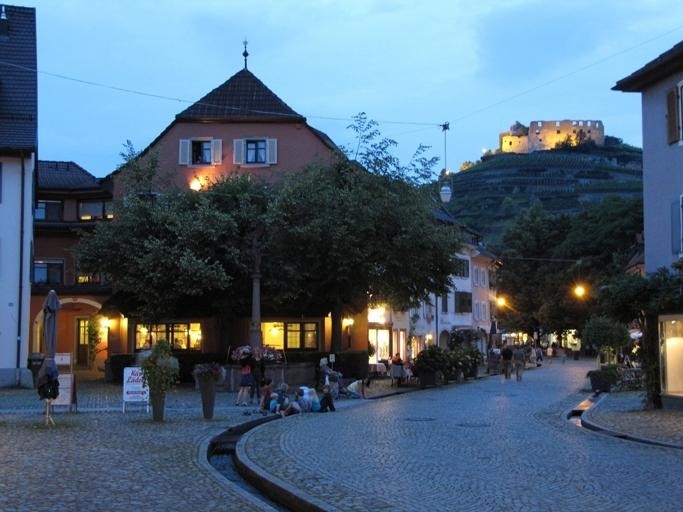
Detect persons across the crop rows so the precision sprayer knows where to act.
[386,353,411,369]
[346,375,369,399]
[173,338,186,350]
[498,336,580,383]
[232,347,350,415]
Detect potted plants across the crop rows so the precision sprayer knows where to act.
[585,365,613,392]
[192,358,224,420]
[137,334,181,421]
[410,343,486,389]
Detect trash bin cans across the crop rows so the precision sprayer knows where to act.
[574,351,579,360]
[28,353,44,386]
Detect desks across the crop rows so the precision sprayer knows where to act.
[368,359,418,388]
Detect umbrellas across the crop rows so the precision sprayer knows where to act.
[34,288,61,424]
